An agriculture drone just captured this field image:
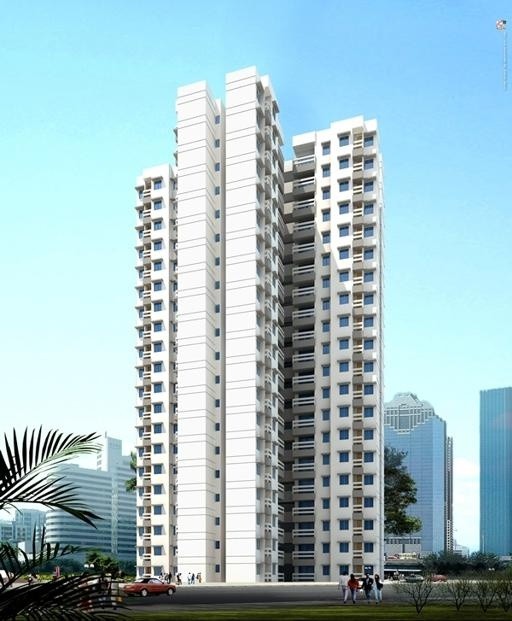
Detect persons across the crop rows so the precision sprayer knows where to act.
[373,574,384,605]
[359,575,374,604]
[158,573,202,586]
[338,571,350,605]
[357,578,365,601]
[347,574,359,605]
[82,567,89,588]
